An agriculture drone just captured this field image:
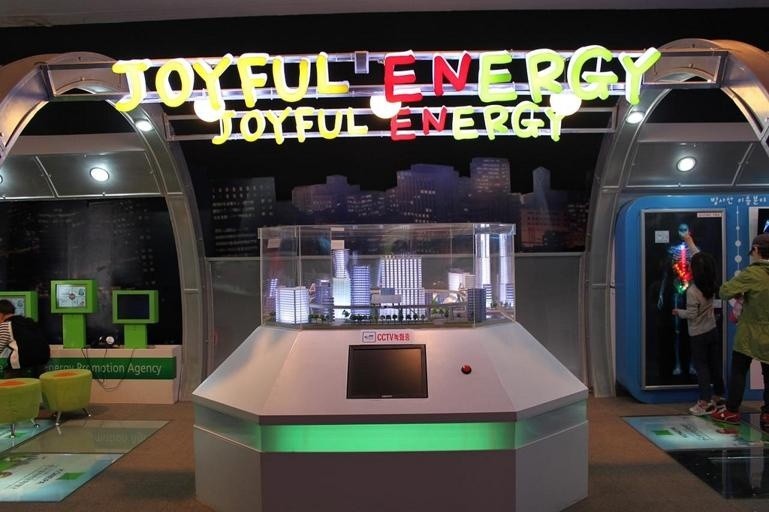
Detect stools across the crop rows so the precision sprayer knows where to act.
[0,368,92,439]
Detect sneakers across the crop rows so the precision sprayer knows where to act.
[712,405,743,425]
[759,411,769,428]
[687,396,726,417]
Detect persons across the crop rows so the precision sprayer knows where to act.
[0,300,50,379]
[673,233,726,415]
[712,234,769,430]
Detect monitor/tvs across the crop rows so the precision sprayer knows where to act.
[51,281,97,313]
[112,290,158,323]
[347,344,429,399]
[0,291,38,322]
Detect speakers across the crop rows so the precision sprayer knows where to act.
[354,51,369,75]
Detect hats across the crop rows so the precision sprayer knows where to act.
[747,233,769,257]
